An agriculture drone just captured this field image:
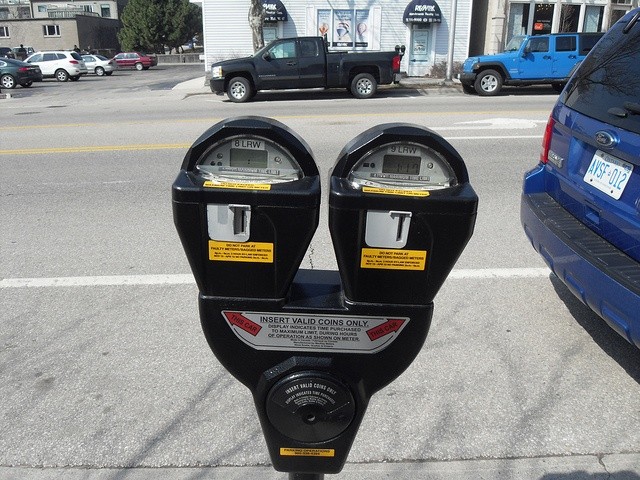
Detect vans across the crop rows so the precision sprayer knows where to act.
[22,50,87,83]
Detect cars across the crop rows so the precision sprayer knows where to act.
[113,52,158,70]
[81,54,115,76]
[0,59,43,88]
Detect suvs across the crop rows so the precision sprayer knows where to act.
[519,6,640,348]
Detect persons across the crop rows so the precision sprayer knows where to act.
[73,45,80,52]
[19,44,26,52]
[88,46,94,54]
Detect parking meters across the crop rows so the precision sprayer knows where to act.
[170,115,481,480]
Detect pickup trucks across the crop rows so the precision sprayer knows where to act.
[460,32,609,96]
[209,34,406,102]
